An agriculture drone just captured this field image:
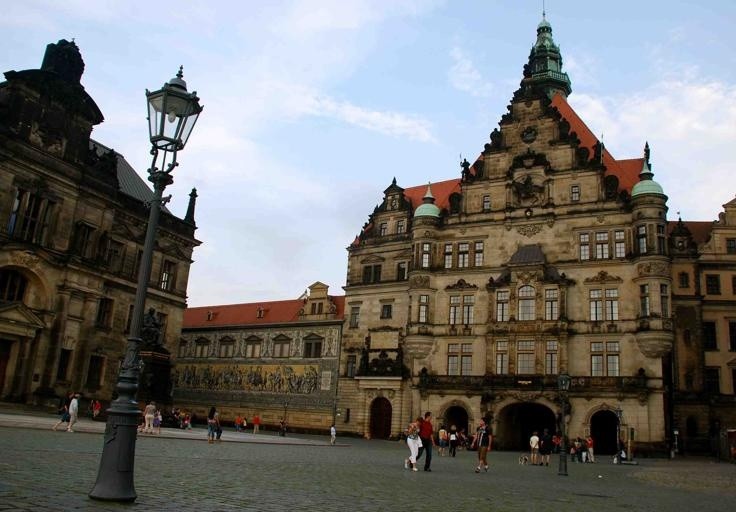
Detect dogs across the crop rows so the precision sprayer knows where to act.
[519,453,528,464]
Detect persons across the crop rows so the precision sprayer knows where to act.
[139,401,192,435]
[405,412,433,472]
[207,407,222,442]
[471,417,492,472]
[330,424,336,445]
[438,424,469,457]
[235,415,261,434]
[280,418,287,432]
[53,392,81,433]
[530,429,594,466]
[592,140,604,160]
[88,400,101,418]
[612,447,627,464]
[461,158,470,181]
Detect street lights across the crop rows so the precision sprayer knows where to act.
[84,66,203,505]
[280,399,288,435]
[613,405,623,465]
[556,372,570,477]
